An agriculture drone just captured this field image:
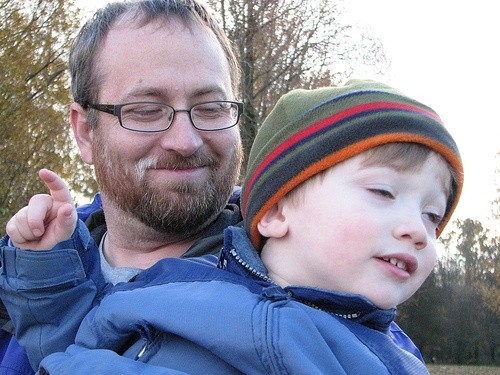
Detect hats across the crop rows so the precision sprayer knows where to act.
[240,80,464,252]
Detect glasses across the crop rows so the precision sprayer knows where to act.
[84,101,244,132]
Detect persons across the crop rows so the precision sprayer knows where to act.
[0,0,241,375]
[0,79,464,375]
